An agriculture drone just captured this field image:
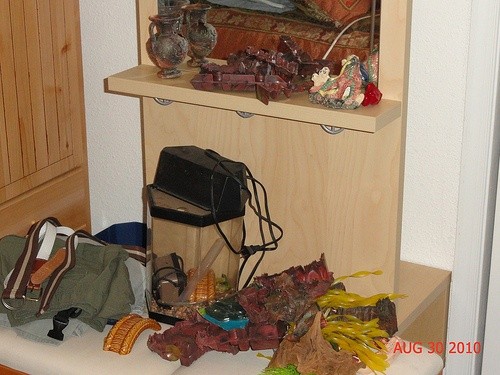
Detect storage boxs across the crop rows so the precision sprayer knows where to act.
[143,185,247,324]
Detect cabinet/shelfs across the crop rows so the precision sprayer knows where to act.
[1,0,95,237]
[102,2,412,313]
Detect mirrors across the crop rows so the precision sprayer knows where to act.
[154,0,379,91]
